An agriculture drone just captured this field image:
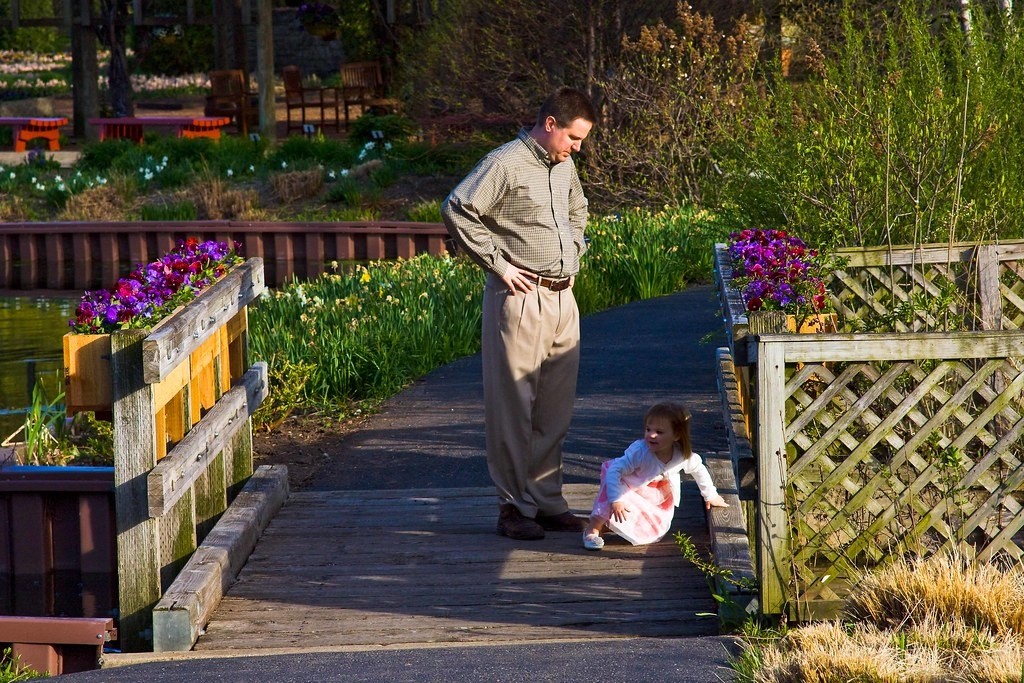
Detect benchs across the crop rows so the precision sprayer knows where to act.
[87,117,230,145]
[0,118,69,151]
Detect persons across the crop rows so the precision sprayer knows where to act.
[582,402,731,548]
[441,87,599,540]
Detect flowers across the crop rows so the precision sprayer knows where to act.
[728,230,831,312]
[70,236,242,330]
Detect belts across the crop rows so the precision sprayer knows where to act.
[519,272,571,291]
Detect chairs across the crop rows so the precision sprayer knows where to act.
[339,61,400,134]
[283,65,339,137]
[204,69,259,136]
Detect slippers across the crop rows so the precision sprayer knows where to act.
[582,531,604,549]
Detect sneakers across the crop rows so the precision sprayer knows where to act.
[496,503,545,540]
[535,509,586,532]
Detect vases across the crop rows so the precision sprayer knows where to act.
[751,310,839,334]
[63,306,248,458]
